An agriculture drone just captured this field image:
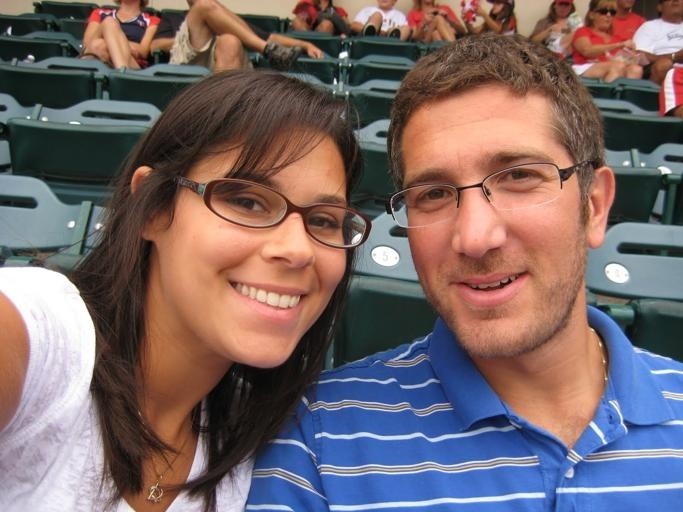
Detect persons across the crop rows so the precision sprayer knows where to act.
[531,1,682,119]
[290,0,518,47]
[244,36,682,511]
[1,70,372,512]
[84,1,323,76]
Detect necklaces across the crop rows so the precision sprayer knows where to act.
[130,399,197,503]
[587,326,611,402]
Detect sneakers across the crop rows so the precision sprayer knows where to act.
[388,27,400,39]
[362,23,376,36]
[263,40,301,71]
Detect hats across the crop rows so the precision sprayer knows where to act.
[486,0,514,7]
[556,0,574,11]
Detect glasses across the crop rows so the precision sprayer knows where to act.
[594,7,616,16]
[172,174,372,250]
[384,158,599,230]
